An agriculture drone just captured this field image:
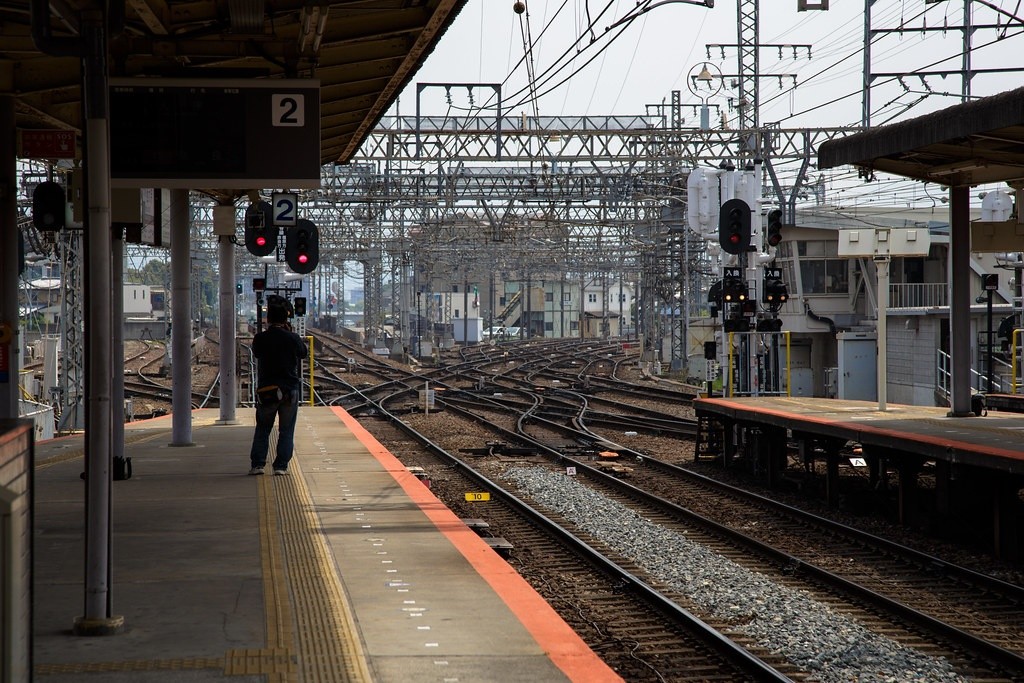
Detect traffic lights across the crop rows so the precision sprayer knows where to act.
[718,198,751,255]
[703,341,716,360]
[33,181,66,234]
[236,284,243,294]
[724,318,750,333]
[767,208,782,246]
[757,319,782,332]
[285,219,320,275]
[295,296,307,314]
[980,273,999,290]
[245,201,278,257]
[763,278,788,304]
[724,278,747,302]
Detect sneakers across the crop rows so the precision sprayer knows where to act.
[248,467,264,475]
[274,469,290,475]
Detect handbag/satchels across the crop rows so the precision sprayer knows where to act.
[255,384,284,406]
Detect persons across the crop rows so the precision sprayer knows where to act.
[248,305,307,476]
